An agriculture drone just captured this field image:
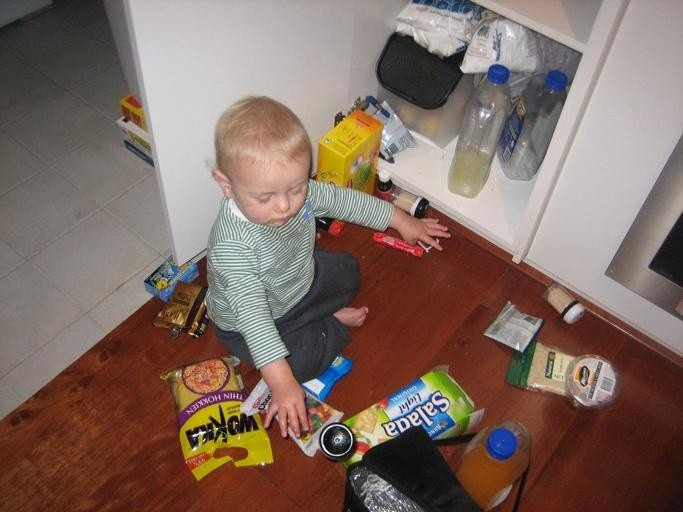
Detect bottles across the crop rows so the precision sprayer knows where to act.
[376,171,430,220]
[542,282,586,326]
[315,216,344,237]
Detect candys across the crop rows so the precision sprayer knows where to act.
[287,397,333,443]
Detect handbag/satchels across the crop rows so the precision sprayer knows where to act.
[342,424,485,512]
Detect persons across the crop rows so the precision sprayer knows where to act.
[201,96,453,441]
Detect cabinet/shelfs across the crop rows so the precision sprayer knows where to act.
[356,0,630,266]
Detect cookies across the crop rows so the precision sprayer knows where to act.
[354,410,378,433]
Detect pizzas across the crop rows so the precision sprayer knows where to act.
[183,359,228,393]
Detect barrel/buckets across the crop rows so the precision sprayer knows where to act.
[496,71,567,180]
[447,63,511,198]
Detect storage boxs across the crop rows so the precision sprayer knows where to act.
[378,31,473,149]
